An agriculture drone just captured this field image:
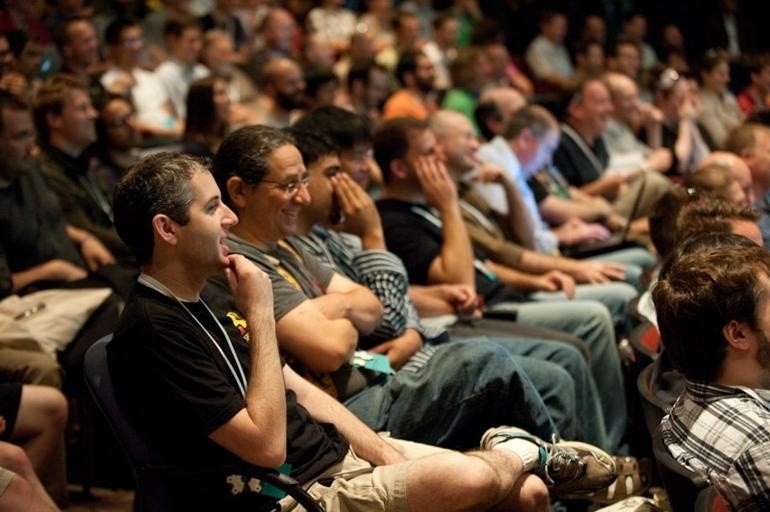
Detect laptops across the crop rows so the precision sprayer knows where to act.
[568,171,646,260]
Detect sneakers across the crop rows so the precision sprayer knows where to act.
[479,426,616,492]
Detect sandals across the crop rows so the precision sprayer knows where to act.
[564,455,649,503]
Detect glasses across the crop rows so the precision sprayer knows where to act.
[262,177,311,192]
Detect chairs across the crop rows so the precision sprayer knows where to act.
[0,0,770,512]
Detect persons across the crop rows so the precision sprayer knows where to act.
[105,151,637,512]
[162,4,769,192]
[651,244,769,512]
[637,123,768,401]
[0,2,186,512]
